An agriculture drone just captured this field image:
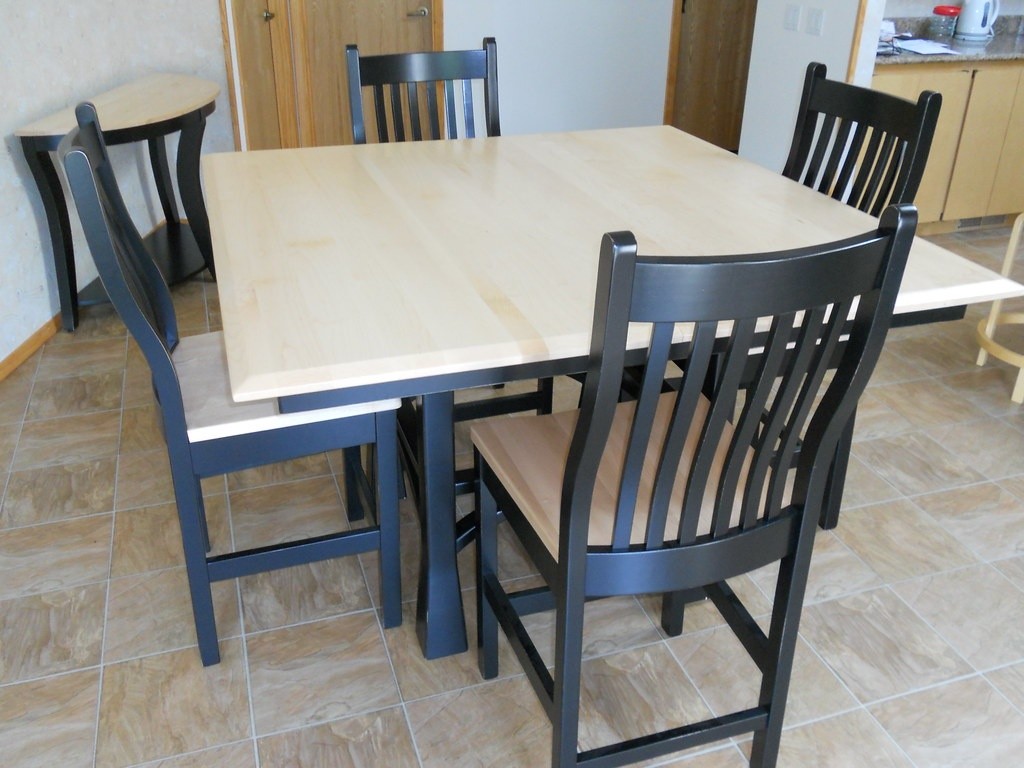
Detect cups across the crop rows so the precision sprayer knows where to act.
[928,6,959,42]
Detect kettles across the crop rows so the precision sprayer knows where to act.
[955,0,1001,40]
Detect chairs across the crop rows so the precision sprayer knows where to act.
[56,97,403,665]
[470,205,920,768]
[346,38,500,145]
[615,63,943,533]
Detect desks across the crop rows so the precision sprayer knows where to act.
[16,74,220,333]
[200,126,1024,658]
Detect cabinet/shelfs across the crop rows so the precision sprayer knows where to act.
[858,60,1024,224]
